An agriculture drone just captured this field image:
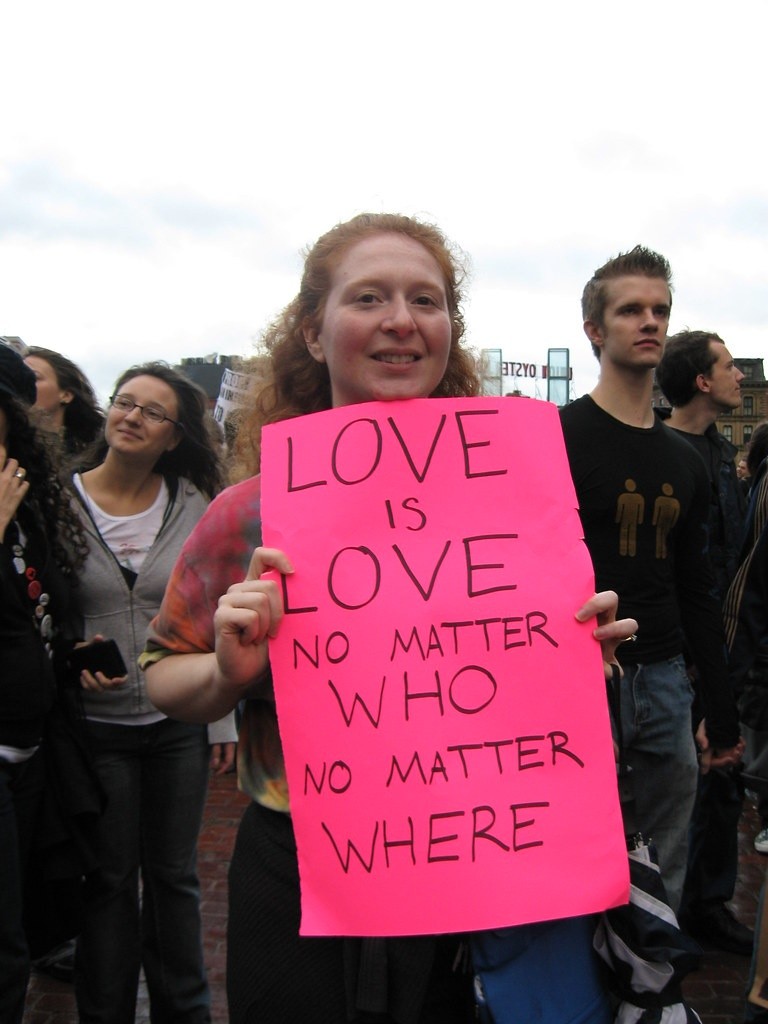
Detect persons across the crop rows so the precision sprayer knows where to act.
[0,212,768,1024]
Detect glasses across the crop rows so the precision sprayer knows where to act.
[110,395,183,430]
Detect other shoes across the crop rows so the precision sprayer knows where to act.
[671,902,753,976]
[38,942,77,980]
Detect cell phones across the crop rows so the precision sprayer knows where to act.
[69,639,128,682]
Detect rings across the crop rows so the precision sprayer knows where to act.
[616,634,637,641]
[15,471,24,480]
